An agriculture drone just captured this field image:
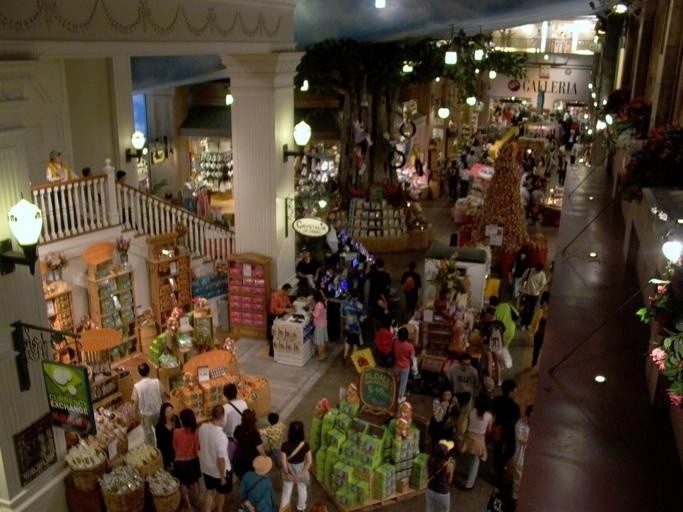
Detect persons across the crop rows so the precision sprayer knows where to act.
[267,250,422,367]
[438,101,581,226]
[130,363,312,511]
[46,150,133,231]
[361,244,555,511]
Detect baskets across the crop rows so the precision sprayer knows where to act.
[124,447,165,483]
[147,477,182,512]
[65,446,109,492]
[99,486,146,512]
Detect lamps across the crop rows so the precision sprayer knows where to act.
[613,0,633,14]
[474,26,489,62]
[437,78,450,119]
[444,22,458,66]
[282,120,311,163]
[0,193,42,277]
[125,130,146,163]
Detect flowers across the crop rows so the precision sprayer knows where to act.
[613,100,652,140]
[600,88,630,115]
[637,253,683,419]
[617,123,683,202]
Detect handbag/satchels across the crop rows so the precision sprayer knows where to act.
[237,498,257,511]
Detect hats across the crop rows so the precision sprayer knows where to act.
[251,454,272,477]
[437,438,455,455]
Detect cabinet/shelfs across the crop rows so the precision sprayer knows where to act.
[227,253,271,340]
[44,279,78,365]
[86,266,142,370]
[145,253,192,333]
